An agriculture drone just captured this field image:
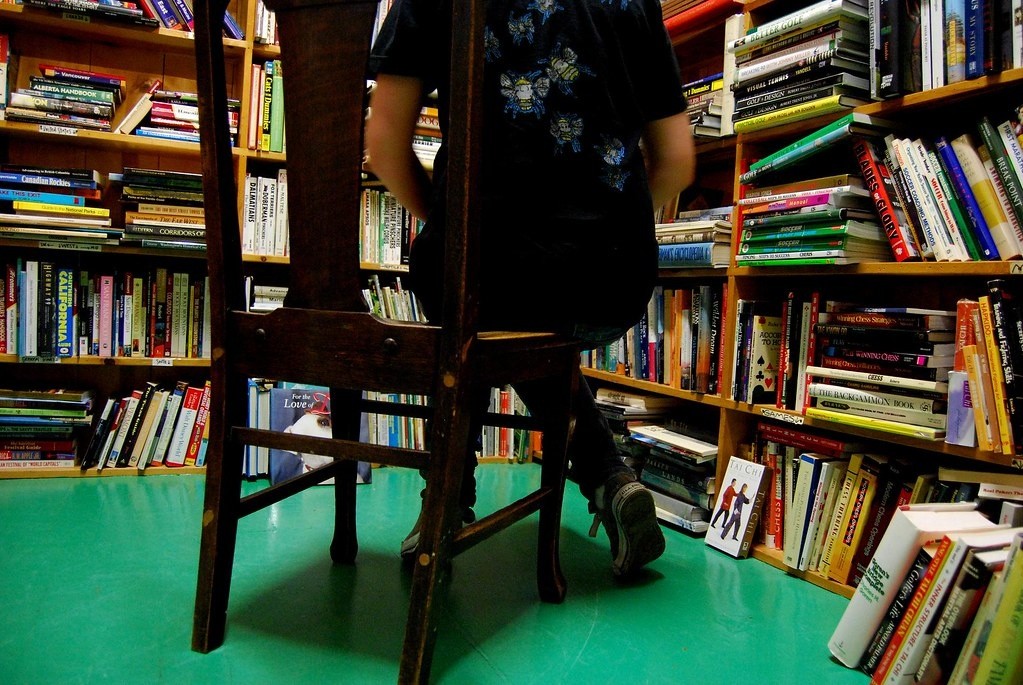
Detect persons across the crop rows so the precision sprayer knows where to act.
[710,479,739,529]
[721,483,755,541]
[360,0,696,578]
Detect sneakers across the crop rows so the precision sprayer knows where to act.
[590,467,666,583]
[400,488,475,560]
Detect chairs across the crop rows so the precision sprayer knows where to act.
[191,0,582,685]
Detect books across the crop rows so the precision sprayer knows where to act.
[704,453,774,558]
[0,0,1023,685]
[270,386,372,489]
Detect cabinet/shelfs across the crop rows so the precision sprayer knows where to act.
[0,1,1023,597]
